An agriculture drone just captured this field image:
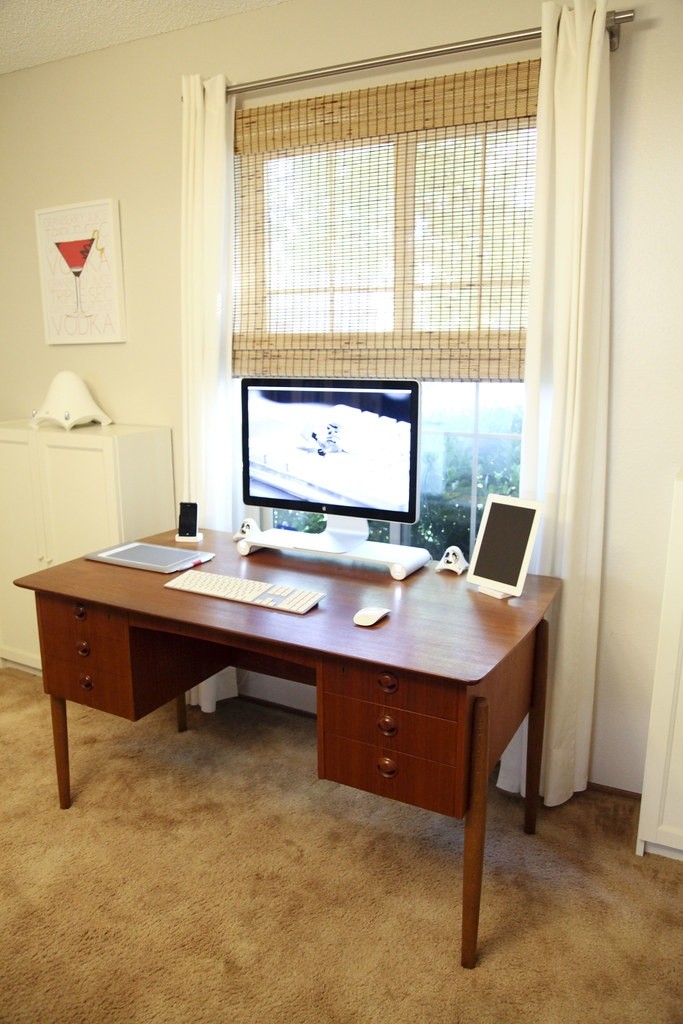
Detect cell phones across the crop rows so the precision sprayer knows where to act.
[179,502,197,537]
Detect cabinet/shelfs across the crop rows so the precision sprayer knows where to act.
[1,418,177,678]
[317,655,470,819]
[34,589,228,722]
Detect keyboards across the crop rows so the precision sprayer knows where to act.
[164,570,327,615]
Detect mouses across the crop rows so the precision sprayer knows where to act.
[353,607,391,626]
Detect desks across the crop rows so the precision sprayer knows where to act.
[13,528,565,969]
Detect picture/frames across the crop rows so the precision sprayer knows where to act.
[32,197,128,346]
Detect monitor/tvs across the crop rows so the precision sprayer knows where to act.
[241,376,422,553]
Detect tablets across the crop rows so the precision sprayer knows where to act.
[465,494,543,597]
